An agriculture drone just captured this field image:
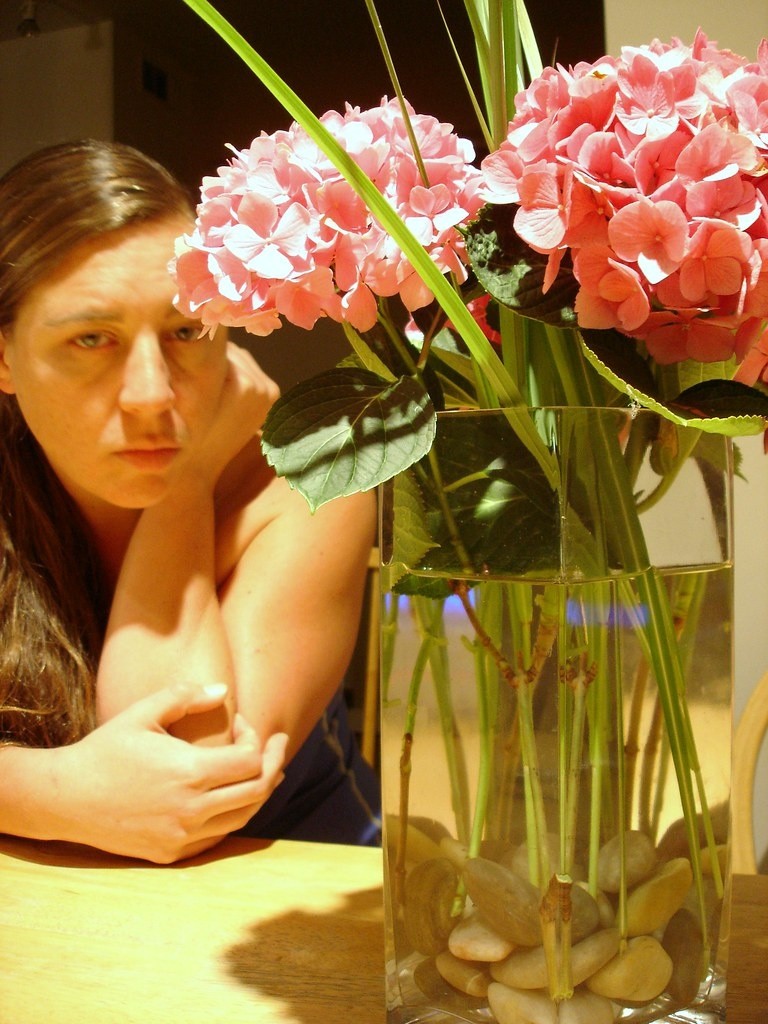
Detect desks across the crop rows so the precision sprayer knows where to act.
[0,835,768,1024]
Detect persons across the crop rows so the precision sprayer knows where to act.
[0,139,383,865]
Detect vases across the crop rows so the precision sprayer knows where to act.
[378,403,735,1024]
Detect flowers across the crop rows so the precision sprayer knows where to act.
[163,0,768,1001]
